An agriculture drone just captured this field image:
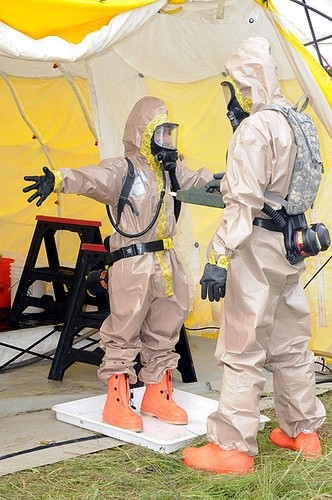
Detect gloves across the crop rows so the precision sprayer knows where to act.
[22,166,56,207]
[198,262,227,302]
[205,172,226,193]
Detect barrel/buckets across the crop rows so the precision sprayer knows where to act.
[0,258,15,308]
[7,263,47,314]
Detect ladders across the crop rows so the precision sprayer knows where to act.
[48,243,198,383]
[8,215,103,322]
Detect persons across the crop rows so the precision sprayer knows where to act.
[22,96,216,432]
[181,39,327,474]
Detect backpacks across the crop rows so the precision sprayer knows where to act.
[254,94,325,217]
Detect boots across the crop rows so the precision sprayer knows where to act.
[101,373,143,432]
[140,369,188,425]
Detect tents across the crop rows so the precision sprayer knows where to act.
[0,0,332,378]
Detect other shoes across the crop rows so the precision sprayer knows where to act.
[270,426,322,458]
[183,436,255,477]
